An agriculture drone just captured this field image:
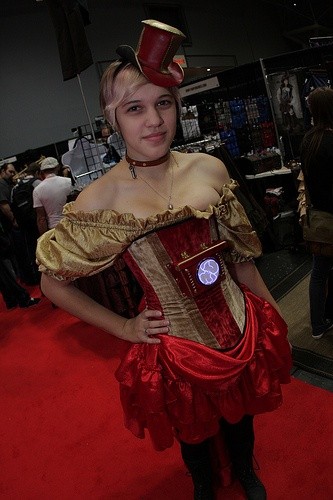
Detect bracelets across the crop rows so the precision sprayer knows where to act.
[120,320,128,337]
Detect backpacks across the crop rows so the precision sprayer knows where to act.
[10,177,38,228]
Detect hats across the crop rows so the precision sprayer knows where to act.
[40,157,59,171]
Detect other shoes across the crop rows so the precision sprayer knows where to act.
[21,298,40,308]
[312,318,333,340]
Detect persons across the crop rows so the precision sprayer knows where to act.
[299,88,333,339]
[0,157,74,309]
[277,75,297,119]
[36,20,293,500]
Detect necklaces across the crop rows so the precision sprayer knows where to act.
[125,150,175,210]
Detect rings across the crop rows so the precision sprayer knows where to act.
[145,329,148,335]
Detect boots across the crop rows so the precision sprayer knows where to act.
[219,415,266,500]
[180,438,216,500]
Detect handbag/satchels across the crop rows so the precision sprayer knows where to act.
[303,210,333,245]
